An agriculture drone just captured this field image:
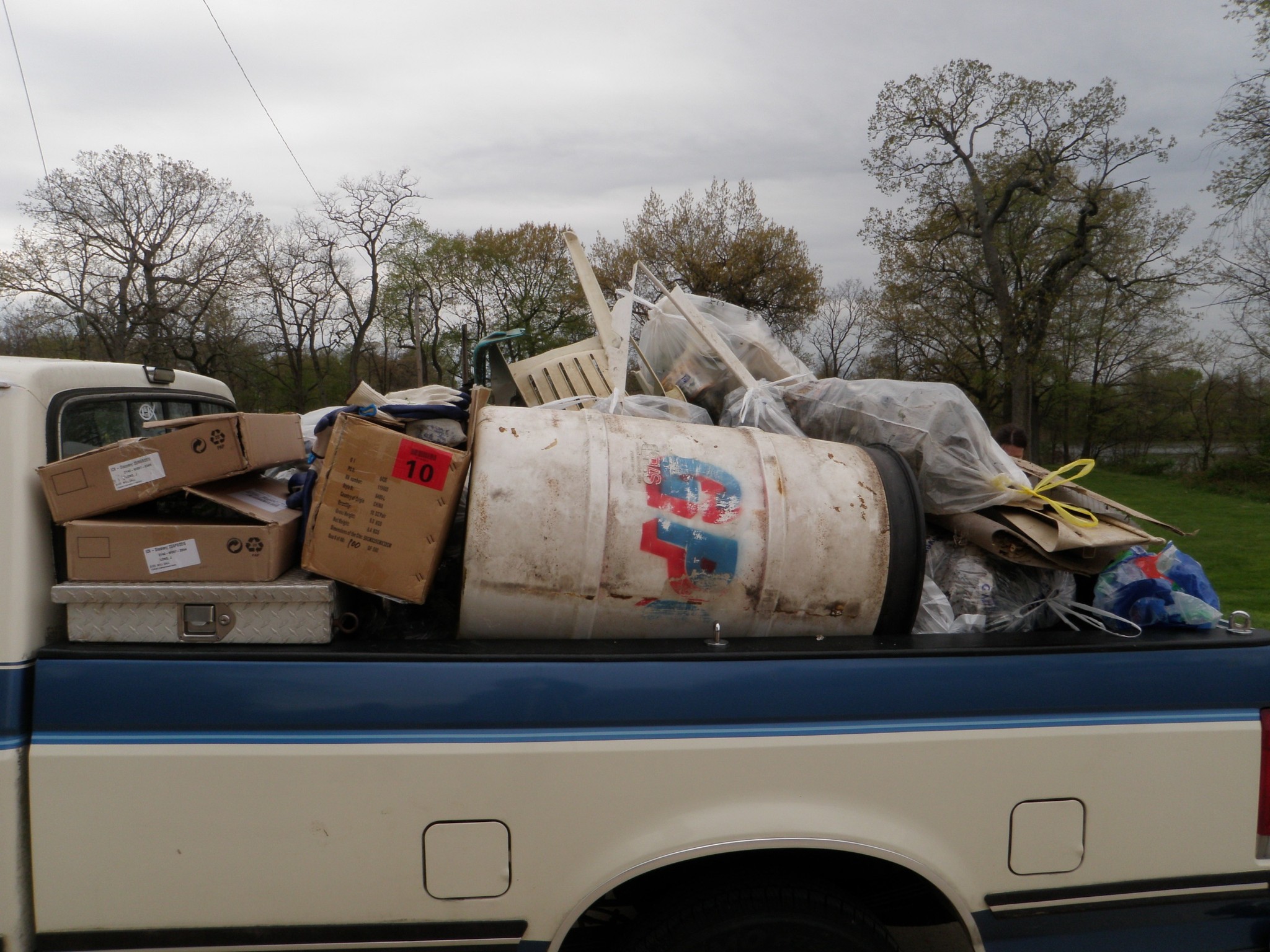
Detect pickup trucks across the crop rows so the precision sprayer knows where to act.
[0,354,1270,951]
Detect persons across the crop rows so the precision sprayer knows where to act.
[990,424,1028,460]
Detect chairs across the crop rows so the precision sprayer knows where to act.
[488,227,632,410]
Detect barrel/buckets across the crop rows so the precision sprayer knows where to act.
[457,404,927,641]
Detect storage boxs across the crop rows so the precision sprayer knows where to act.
[302,405,470,606]
[62,483,298,585]
[37,414,299,523]
[51,577,335,643]
